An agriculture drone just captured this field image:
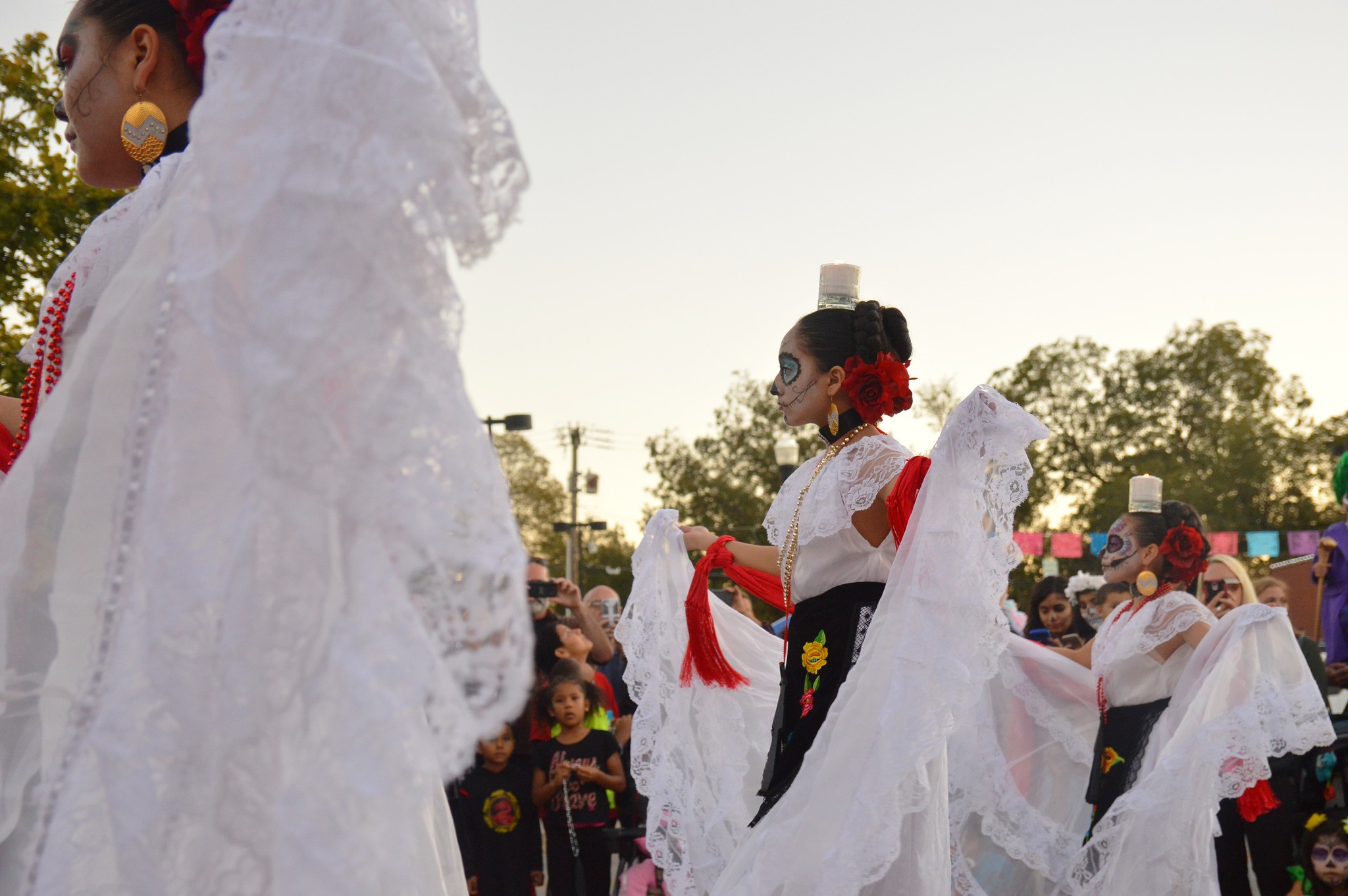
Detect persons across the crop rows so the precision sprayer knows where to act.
[1007,501,1282,896]
[442,553,1131,896]
[0,3,539,895]
[1195,451,1348,896]
[666,300,998,896]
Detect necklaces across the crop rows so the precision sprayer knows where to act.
[777,423,868,606]
[6,271,77,476]
[1096,583,1173,724]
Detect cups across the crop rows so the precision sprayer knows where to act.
[1128,476,1164,515]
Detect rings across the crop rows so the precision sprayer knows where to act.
[1217,606,1222,612]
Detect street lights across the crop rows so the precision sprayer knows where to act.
[555,520,609,599]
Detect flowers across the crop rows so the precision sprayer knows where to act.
[841,354,919,427]
[169,0,231,81]
[1161,522,1213,585]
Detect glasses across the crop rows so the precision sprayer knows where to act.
[1225,579,1241,592]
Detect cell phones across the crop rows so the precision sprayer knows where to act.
[1204,579,1228,609]
[527,580,558,598]
[1062,634,1084,650]
[601,598,621,637]
[1028,629,1056,648]
